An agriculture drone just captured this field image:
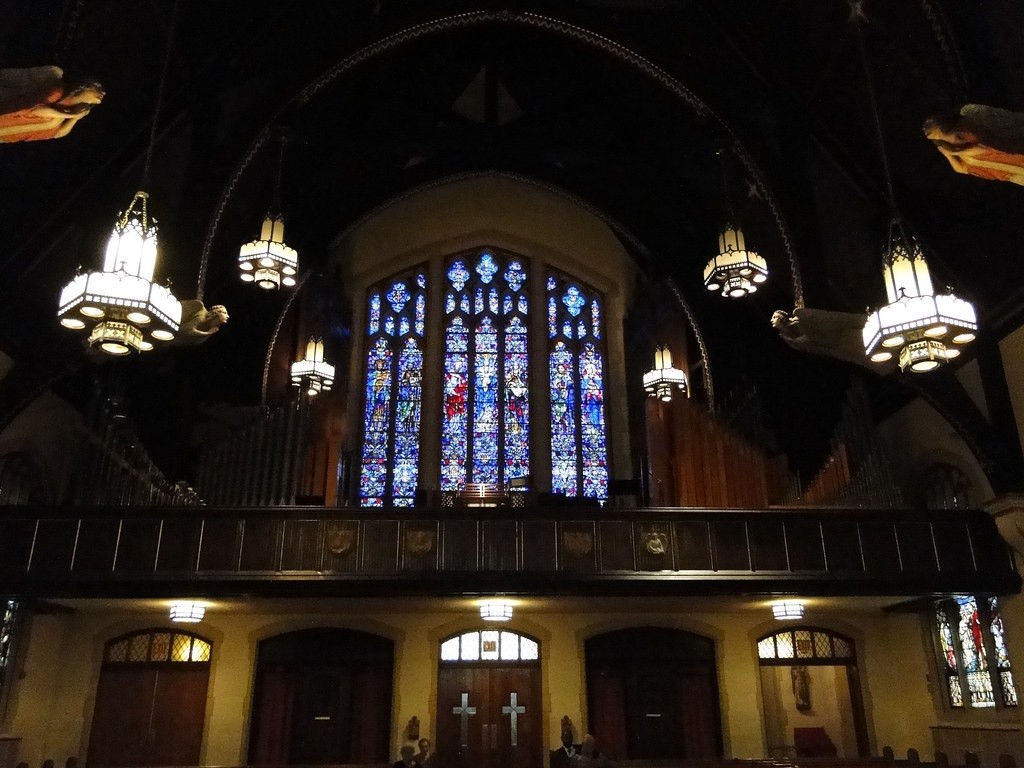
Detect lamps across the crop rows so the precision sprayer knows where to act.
[169,605,206,623]
[851,0,983,373]
[56,0,204,356]
[642,343,689,401]
[772,602,807,622]
[479,604,513,622]
[235,136,301,296]
[291,332,336,397]
[703,208,770,297]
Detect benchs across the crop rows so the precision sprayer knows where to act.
[457,482,509,508]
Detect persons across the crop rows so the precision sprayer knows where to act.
[391,738,435,768]
[549,728,604,768]
